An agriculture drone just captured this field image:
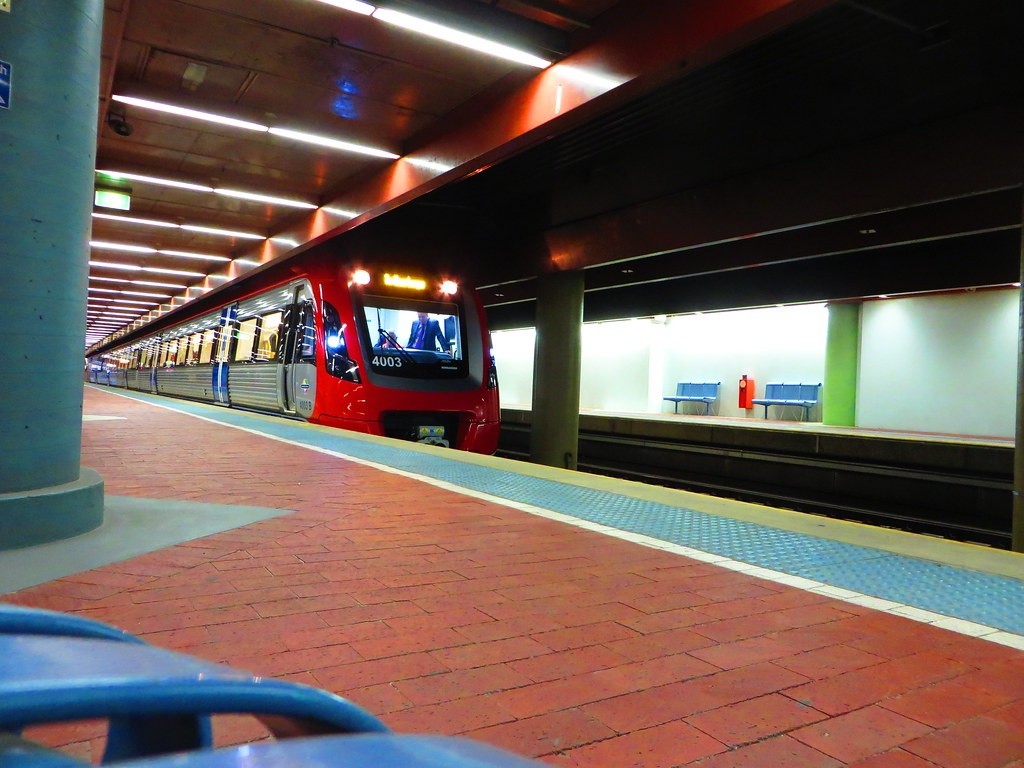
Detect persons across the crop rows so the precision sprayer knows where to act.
[378,332,404,348]
[268,330,279,359]
[406,312,452,355]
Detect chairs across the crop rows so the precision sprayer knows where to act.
[374,334,386,347]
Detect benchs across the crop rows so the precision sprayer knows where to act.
[751,383,822,422]
[663,382,721,416]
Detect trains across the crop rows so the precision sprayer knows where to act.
[88,250,503,457]
